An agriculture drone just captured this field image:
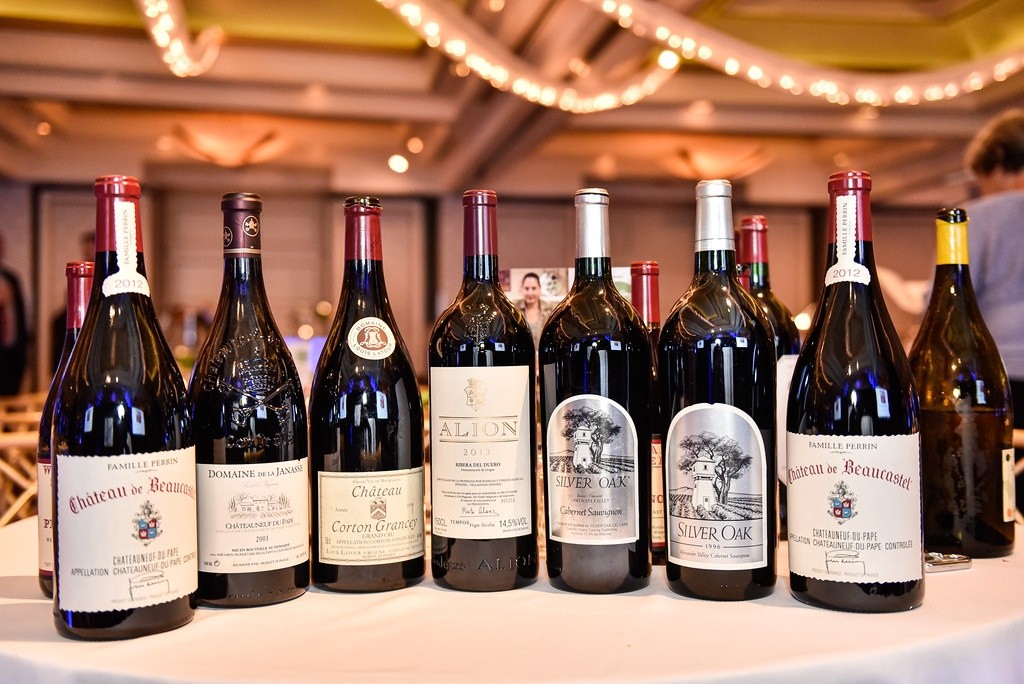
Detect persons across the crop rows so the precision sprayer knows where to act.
[0,233,28,400]
[513,273,553,349]
[955,110,1024,516]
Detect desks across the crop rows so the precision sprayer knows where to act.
[0,490,1024,684]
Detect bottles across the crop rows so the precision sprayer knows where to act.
[907,206,1017,562]
[429,188,541,595]
[307,197,428,594]
[37,263,95,600]
[537,188,655,595]
[181,192,313,609]
[48,173,199,640]
[624,260,669,570]
[657,176,781,602]
[783,169,928,614]
[736,212,803,544]
